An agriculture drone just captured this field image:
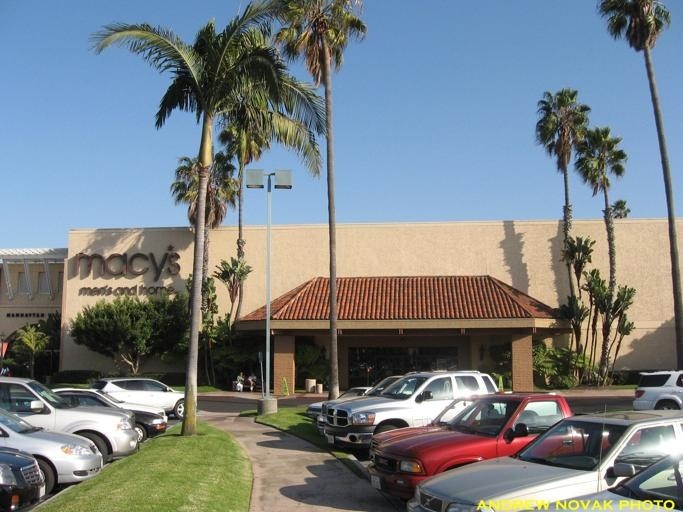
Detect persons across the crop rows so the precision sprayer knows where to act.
[246,371,258,393]
[236,371,246,392]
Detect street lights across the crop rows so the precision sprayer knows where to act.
[246,168,293,399]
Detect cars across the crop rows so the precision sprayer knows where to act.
[307,368,682,512]
[0,375,197,512]
